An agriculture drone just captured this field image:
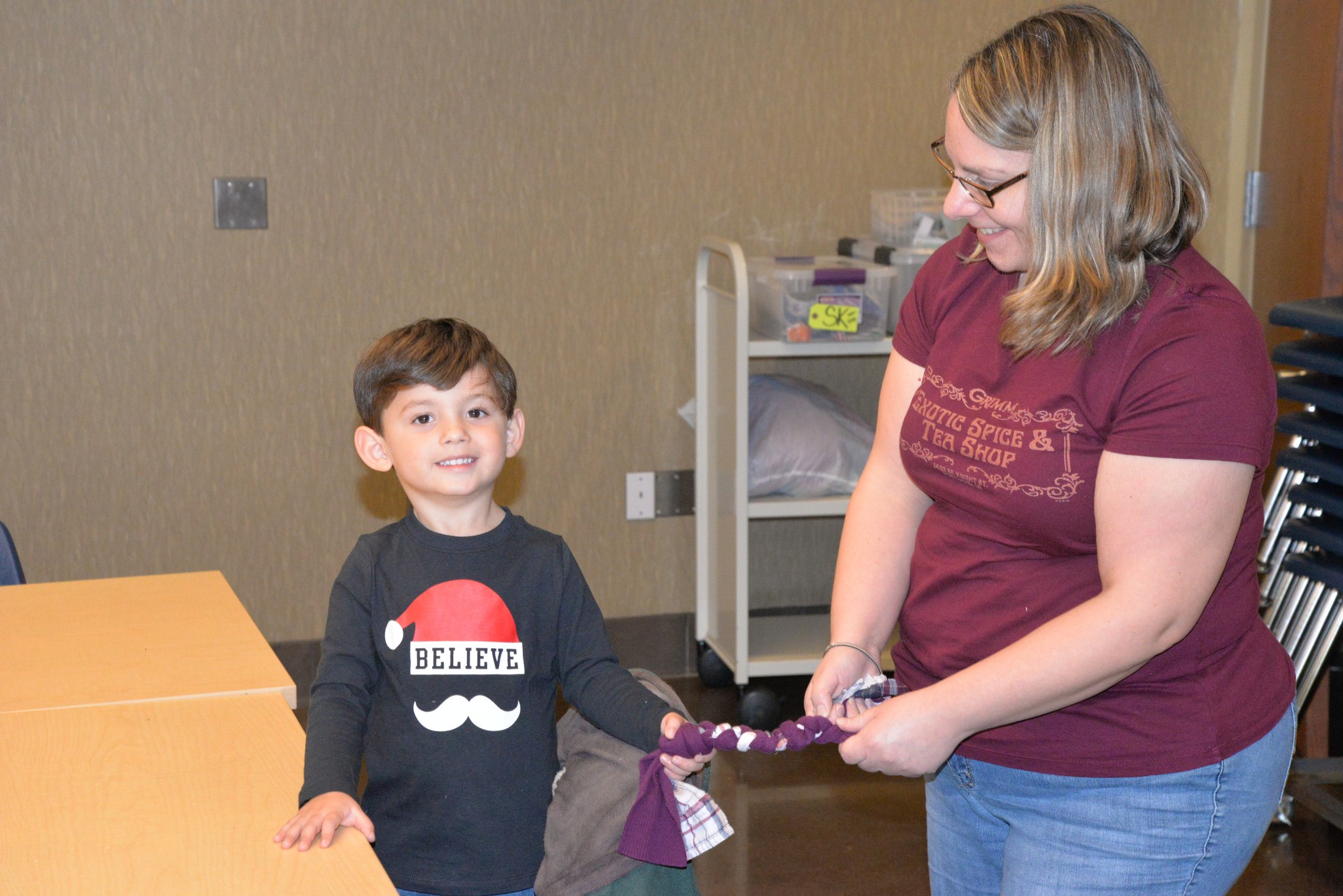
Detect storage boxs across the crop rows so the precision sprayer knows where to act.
[750,252,898,342]
[870,189,967,244]
[837,236,937,336]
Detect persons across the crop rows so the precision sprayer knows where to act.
[271,318,716,896]
[800,6,1298,896]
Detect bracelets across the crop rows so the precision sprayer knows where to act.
[823,642,883,677]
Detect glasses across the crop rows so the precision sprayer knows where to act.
[932,136,1029,209]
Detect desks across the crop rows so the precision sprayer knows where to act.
[0,571,296,713]
[0,692,401,896]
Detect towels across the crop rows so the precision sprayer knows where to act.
[616,714,860,870]
